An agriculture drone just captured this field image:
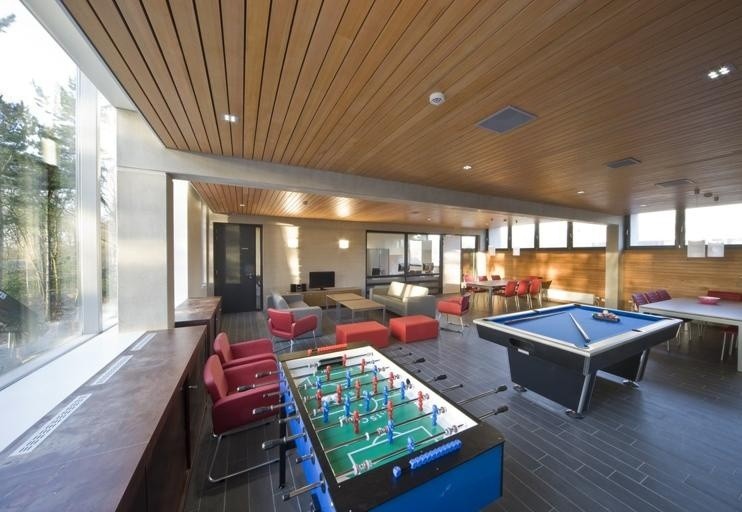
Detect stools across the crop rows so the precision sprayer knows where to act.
[335,320,390,349]
[388,313,440,343]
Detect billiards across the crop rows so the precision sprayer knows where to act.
[593,310,620,323]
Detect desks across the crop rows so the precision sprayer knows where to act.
[472,303,683,416]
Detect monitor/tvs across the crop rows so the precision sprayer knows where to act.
[309,271,335,290]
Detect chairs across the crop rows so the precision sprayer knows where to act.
[632,287,742,373]
[436,294,472,333]
[266,307,319,354]
[203,352,291,484]
[464,272,552,313]
[212,332,278,372]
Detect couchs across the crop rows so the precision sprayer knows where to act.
[368,281,437,321]
[267,290,324,341]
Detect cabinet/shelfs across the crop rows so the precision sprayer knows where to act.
[289,287,363,309]
[1,325,208,512]
[173,295,224,354]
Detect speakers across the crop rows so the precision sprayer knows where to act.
[302,284,306,291]
[290,284,296,292]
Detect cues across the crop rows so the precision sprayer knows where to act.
[569,313,591,342]
[504,311,568,325]
